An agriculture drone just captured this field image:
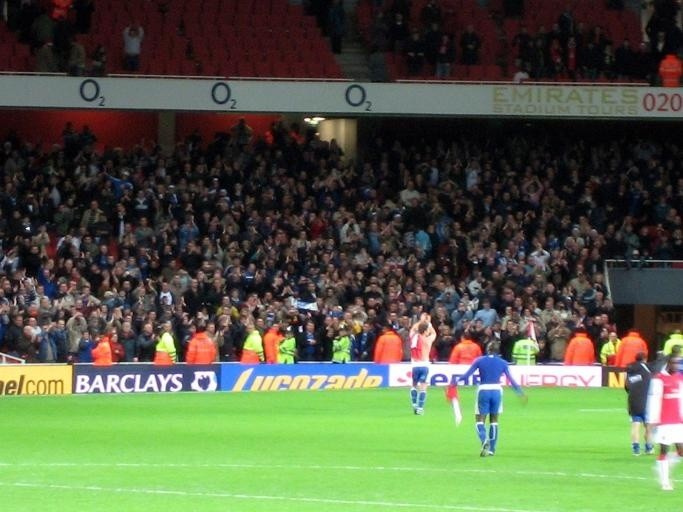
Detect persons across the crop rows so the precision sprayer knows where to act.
[454,340,526,458]
[407,311,438,415]
[625,346,683,493]
[2,1,145,75]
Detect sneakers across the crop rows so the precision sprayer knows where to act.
[633,445,679,490]
[412,404,423,415]
[481,442,495,457]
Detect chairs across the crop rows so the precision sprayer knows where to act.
[0,0,644,85]
